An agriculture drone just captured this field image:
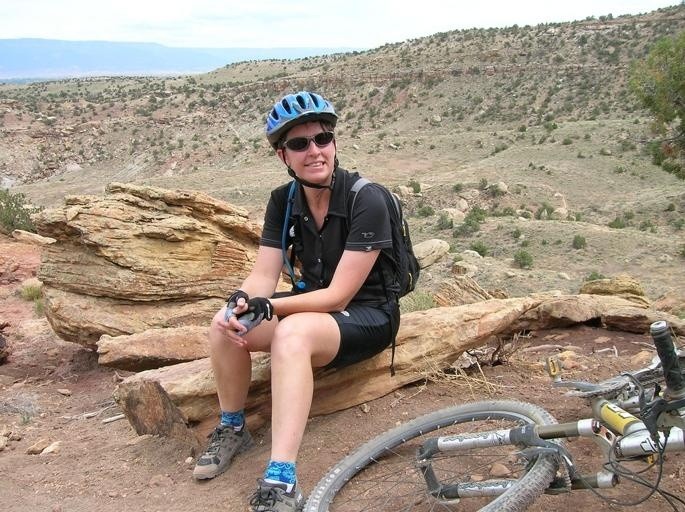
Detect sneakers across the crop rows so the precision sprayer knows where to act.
[193,424,256,482]
[248,478,305,512]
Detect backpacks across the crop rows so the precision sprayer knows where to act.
[346,177,422,303]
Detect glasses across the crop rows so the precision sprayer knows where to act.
[281,131,333,153]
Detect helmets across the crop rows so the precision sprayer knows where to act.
[265,91,338,144]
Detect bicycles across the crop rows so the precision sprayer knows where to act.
[300,320,684,511]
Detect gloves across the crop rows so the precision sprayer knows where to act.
[235,296,273,336]
[225,291,249,322]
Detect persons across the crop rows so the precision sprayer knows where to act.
[190,90,422,512]
